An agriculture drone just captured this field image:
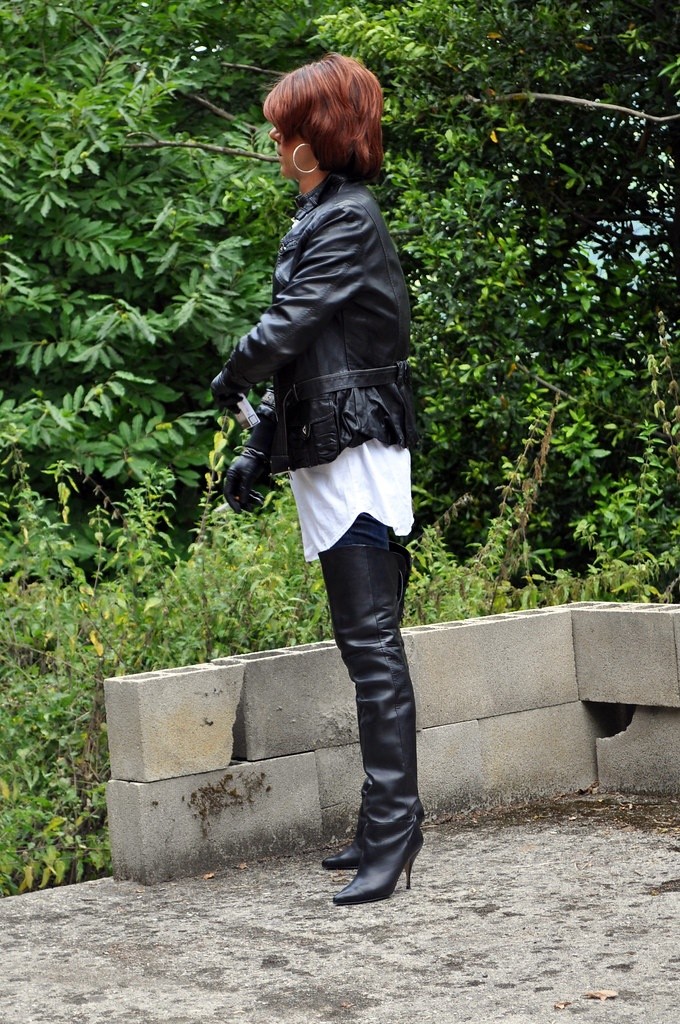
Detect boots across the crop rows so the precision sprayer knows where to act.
[318,541,425,904]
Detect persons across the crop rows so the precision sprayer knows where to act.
[209,53,425,905]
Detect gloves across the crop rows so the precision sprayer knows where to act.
[211,366,253,414]
[224,449,269,515]
